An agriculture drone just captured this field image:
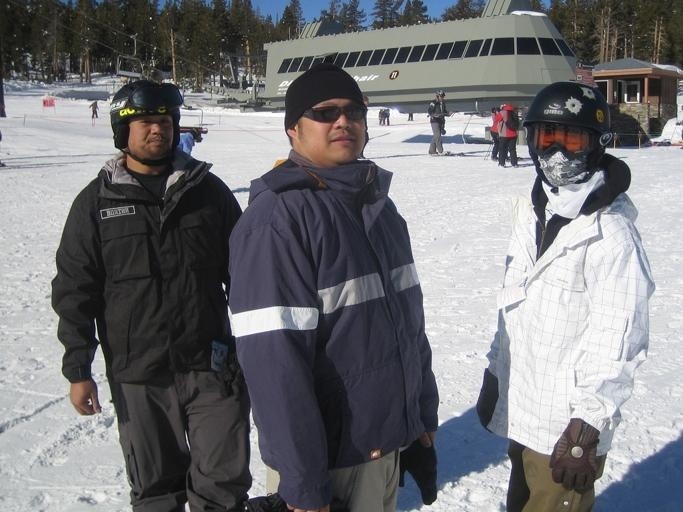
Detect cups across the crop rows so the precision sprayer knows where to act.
[110,82,183,113]
[526,121,601,156]
[301,102,367,123]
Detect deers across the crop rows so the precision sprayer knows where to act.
[535,149,593,188]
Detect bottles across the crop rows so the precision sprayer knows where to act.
[285,62,364,132]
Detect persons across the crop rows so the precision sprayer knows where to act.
[49,79,250,511]
[427,89,453,157]
[222,60,440,512]
[88,101,99,119]
[382,109,389,126]
[490,104,519,169]
[474,80,657,511]
[378,109,383,125]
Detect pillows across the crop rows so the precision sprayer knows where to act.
[436,90,445,97]
[521,82,612,169]
[110,79,183,150]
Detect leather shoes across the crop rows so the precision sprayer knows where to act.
[549,418,599,493]
[400,439,437,504]
[477,367,498,434]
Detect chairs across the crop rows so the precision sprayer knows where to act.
[429,152,448,156]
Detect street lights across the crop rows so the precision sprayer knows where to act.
[502,110,519,131]
